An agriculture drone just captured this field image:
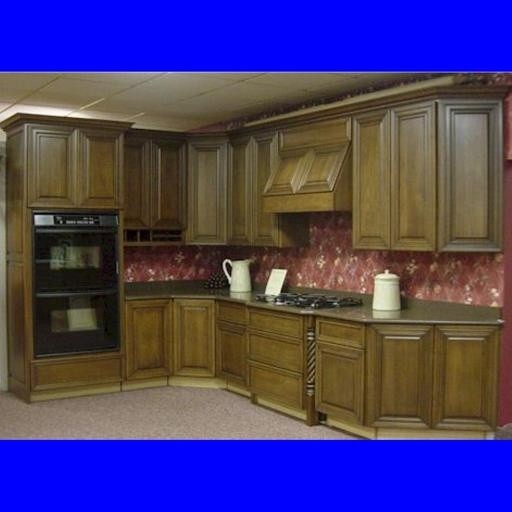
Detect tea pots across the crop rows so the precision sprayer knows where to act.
[221,258,254,293]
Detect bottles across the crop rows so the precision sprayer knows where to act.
[371,269,402,311]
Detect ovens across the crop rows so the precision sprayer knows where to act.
[32,214,121,359]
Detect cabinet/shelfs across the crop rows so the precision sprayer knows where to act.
[172,298,216,388]
[215,299,252,397]
[352,86,436,254]
[245,304,315,427]
[366,321,500,441]
[0,113,135,404]
[314,316,376,439]
[124,299,174,391]
[124,128,187,244]
[437,84,512,252]
[228,108,310,249]
[188,131,228,246]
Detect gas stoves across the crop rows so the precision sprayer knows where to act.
[255,292,362,309]
